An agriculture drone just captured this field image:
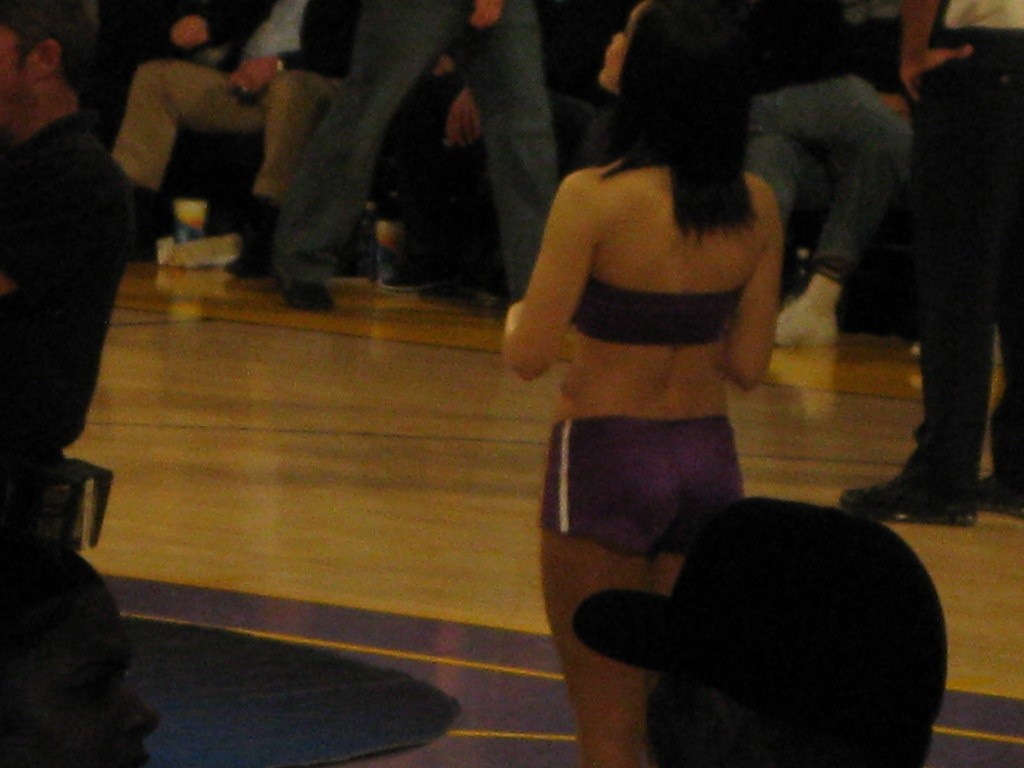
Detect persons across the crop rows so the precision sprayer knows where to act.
[570,495,949,768]
[75,1,925,349]
[0,527,158,767]
[501,1,783,768]
[836,0,1023,526]
[0,0,135,470]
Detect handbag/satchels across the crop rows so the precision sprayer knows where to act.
[835,181,923,339]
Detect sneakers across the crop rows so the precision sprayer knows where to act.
[773,303,839,344]
[840,467,978,525]
[976,472,1024,517]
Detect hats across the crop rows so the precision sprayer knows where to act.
[16,0,98,51]
[570,495,947,729]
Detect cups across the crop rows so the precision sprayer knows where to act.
[374,219,406,289]
[175,200,207,243]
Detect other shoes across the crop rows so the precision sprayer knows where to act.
[274,274,335,309]
[224,245,271,277]
